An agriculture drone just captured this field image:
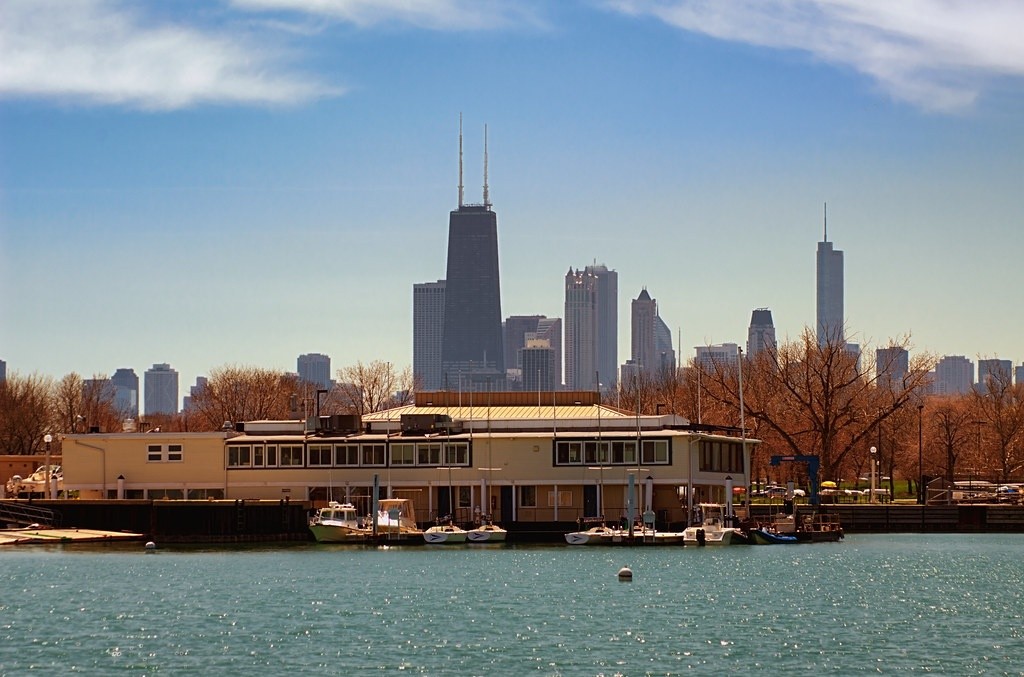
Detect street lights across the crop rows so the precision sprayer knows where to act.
[917,405,926,504]
[971,421,988,480]
[869,446,877,505]
[316,390,329,417]
[43,435,52,500]
[878,407,883,501]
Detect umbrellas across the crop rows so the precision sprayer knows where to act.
[821,481,838,488]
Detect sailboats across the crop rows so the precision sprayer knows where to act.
[562,371,650,545]
[470,392,509,543]
[736,346,795,534]
[420,371,469,544]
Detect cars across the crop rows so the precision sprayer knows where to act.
[735,479,805,499]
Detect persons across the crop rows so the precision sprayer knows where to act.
[854,482,859,503]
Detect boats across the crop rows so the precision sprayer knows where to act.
[734,529,797,546]
[345,498,426,545]
[638,532,684,544]
[307,470,371,543]
[10,465,63,497]
[799,511,845,543]
[681,501,735,546]
